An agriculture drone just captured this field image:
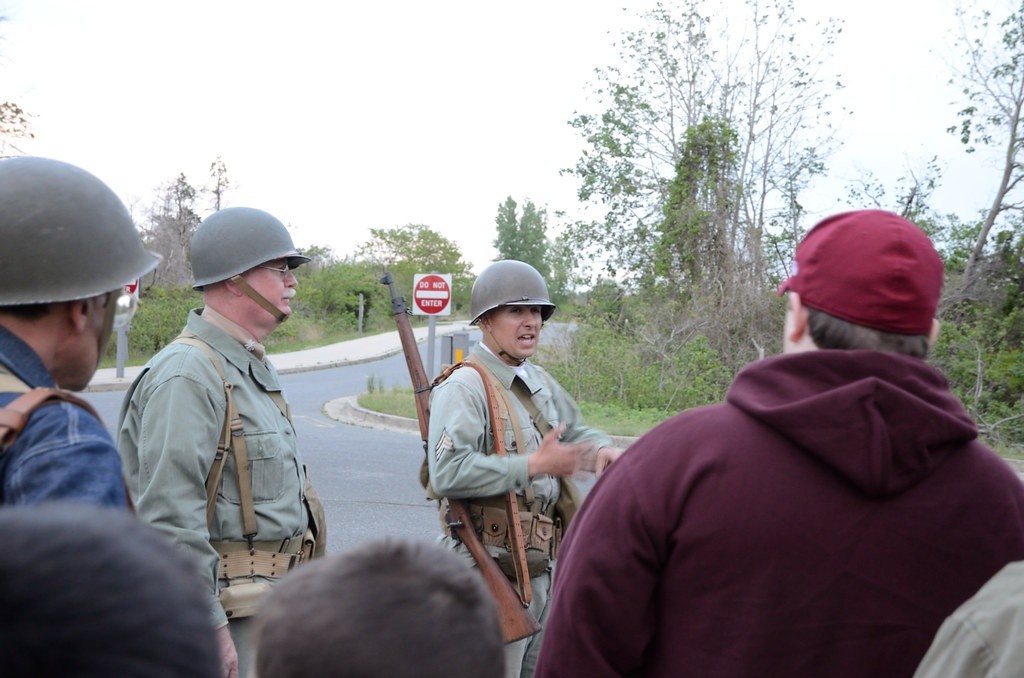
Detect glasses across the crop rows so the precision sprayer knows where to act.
[259,264,289,280]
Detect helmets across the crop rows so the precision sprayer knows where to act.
[0,156,164,303]
[470,260,556,325]
[190,207,311,291]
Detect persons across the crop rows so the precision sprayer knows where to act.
[424,258,629,678]
[114,205,328,678]
[535,207,1024,678]
[0,499,223,678]
[0,155,164,676]
[252,536,504,678]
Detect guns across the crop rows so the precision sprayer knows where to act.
[380,273,542,645]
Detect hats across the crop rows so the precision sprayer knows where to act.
[778,209,944,334]
